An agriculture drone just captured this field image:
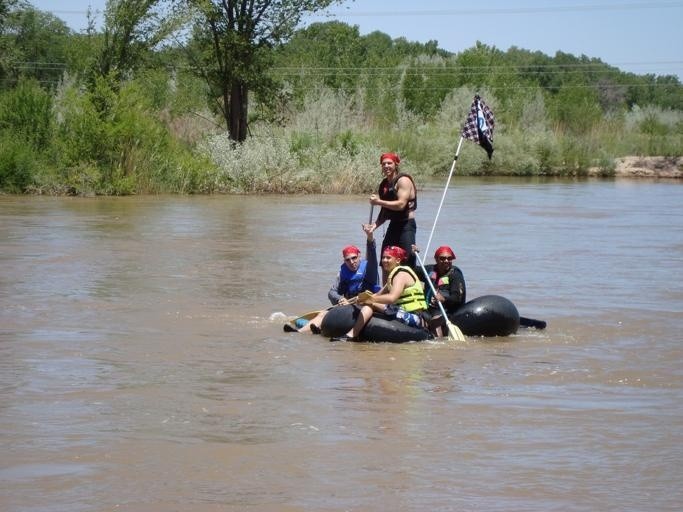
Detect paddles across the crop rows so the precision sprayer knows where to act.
[414,250,465,341]
[290,301,359,325]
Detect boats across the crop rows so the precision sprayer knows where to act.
[317,294,521,341]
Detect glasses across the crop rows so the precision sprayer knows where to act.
[438,257,453,261]
[383,245,395,250]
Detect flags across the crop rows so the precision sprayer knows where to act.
[462,93,495,160]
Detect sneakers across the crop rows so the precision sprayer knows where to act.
[330,335,360,342]
[310,324,321,334]
[284,324,298,332]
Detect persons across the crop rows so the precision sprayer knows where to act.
[369,153,417,288]
[282,223,378,334]
[329,246,430,343]
[404,244,465,341]
